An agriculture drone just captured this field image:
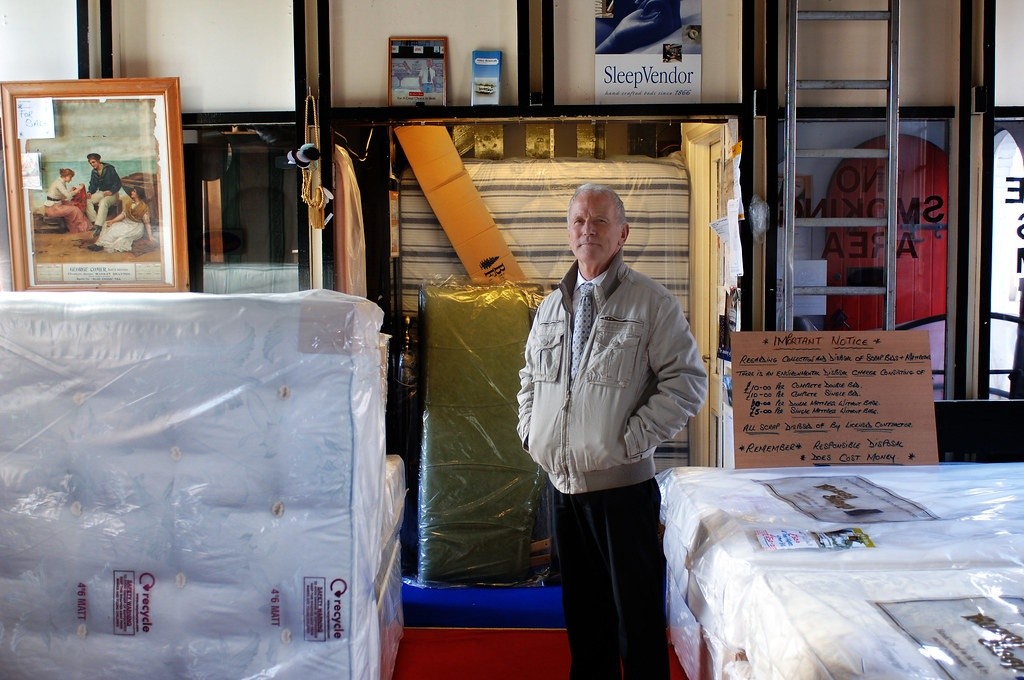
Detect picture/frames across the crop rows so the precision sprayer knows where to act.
[0,77,192,293]
[388,36,447,107]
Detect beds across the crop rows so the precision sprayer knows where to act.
[373,453,406,602]
[739,565,1024,680]
[377,539,405,680]
[662,462,1024,650]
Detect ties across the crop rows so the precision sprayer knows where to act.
[427,67,431,83]
[570,283,595,388]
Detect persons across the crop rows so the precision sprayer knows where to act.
[517,183,706,680]
[418,58,437,94]
